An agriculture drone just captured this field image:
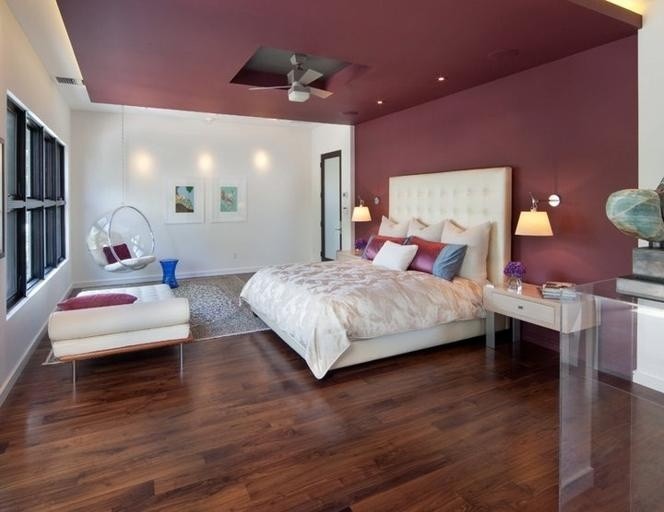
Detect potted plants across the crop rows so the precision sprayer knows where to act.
[504,261,528,292]
[355,237,367,252]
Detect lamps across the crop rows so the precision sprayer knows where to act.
[515,190,560,237]
[350,197,381,224]
[287,86,312,103]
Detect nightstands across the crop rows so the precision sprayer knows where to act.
[483,280,602,371]
[334,248,362,260]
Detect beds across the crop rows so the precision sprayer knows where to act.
[249,213,511,371]
[41,283,195,385]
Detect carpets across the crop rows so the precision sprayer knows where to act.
[81,271,272,344]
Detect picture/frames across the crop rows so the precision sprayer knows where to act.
[163,173,247,225]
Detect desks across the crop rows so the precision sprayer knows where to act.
[158,258,180,287]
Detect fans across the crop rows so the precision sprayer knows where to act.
[248,52,334,100]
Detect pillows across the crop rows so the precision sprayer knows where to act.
[362,214,494,284]
[103,242,133,262]
[57,291,139,311]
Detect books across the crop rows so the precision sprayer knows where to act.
[536,279,577,303]
